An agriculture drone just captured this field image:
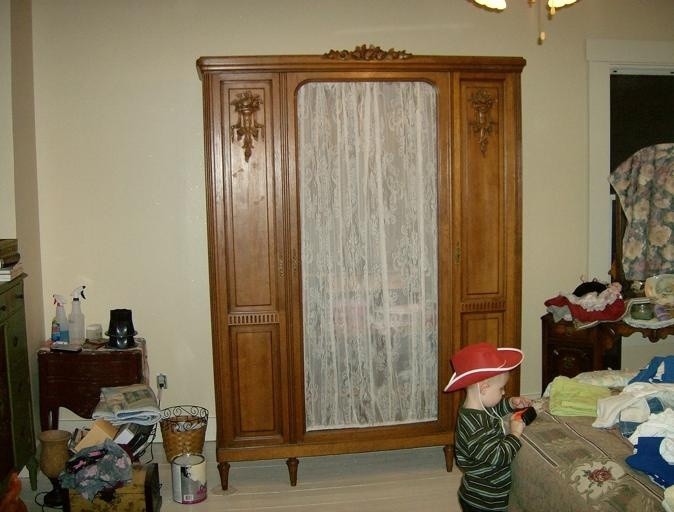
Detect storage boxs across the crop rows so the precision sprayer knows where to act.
[61,463,162,512]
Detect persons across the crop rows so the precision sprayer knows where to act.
[443,343,532,511]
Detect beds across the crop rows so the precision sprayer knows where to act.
[500,386,666,512]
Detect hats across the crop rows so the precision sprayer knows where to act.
[443,342,525,394]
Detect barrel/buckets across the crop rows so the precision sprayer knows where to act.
[171,444,208,504]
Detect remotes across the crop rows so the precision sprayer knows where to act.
[51,344,82,353]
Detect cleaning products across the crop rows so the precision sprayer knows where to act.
[68,284,86,343]
[52,294,68,342]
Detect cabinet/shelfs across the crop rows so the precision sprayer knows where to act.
[39,351,139,432]
[195,58,529,491]
[0,272,37,500]
[540,317,622,399]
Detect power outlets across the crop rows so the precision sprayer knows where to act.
[157,373,167,390]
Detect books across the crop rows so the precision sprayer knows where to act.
[0,238,25,282]
